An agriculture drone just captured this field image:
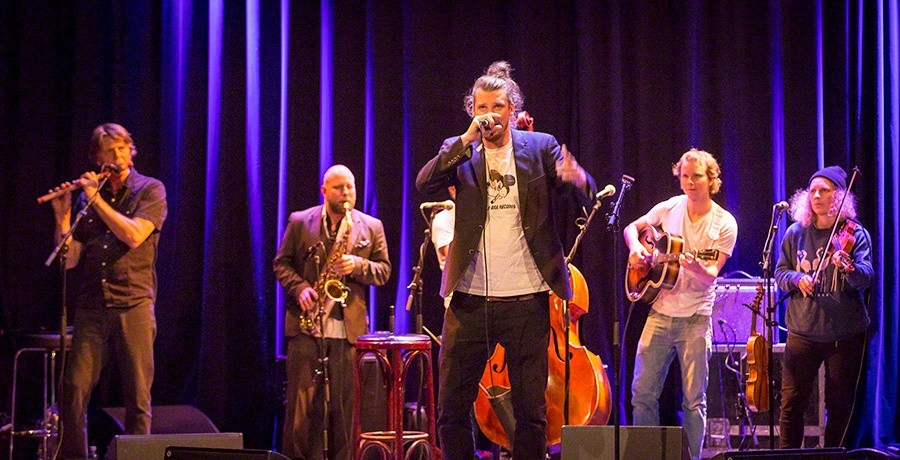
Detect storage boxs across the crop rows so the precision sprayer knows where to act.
[708,344,826,450]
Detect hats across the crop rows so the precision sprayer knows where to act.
[808,166,847,187]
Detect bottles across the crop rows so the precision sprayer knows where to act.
[89,445,99,460]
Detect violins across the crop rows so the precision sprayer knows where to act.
[829,217,859,273]
[745,283,770,415]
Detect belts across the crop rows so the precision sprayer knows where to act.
[485,294,535,301]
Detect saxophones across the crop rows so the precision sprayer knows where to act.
[300,202,355,336]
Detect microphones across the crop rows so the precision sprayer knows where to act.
[420,199,455,211]
[480,119,488,127]
[301,240,320,262]
[773,200,792,212]
[595,183,616,201]
[104,162,124,173]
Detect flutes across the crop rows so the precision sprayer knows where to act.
[36,171,110,205]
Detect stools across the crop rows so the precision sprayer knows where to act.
[349,335,437,460]
[10,327,73,460]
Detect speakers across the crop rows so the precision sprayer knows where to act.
[96,403,291,460]
[710,275,780,342]
[559,423,683,460]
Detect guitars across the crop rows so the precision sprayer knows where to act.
[625,225,722,302]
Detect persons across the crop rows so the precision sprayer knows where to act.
[49,123,168,460]
[273,164,390,460]
[416,62,600,460]
[773,165,874,451]
[623,147,738,460]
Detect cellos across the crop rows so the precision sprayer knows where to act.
[472,258,611,452]
